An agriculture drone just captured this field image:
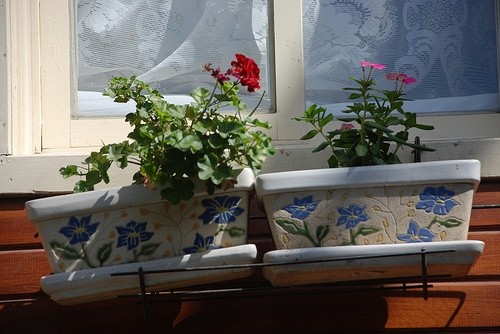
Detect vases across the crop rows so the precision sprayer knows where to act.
[24,165,259,307]
[255,158,483,290]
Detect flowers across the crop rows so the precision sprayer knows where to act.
[58,52,274,206]
[289,60,437,166]
